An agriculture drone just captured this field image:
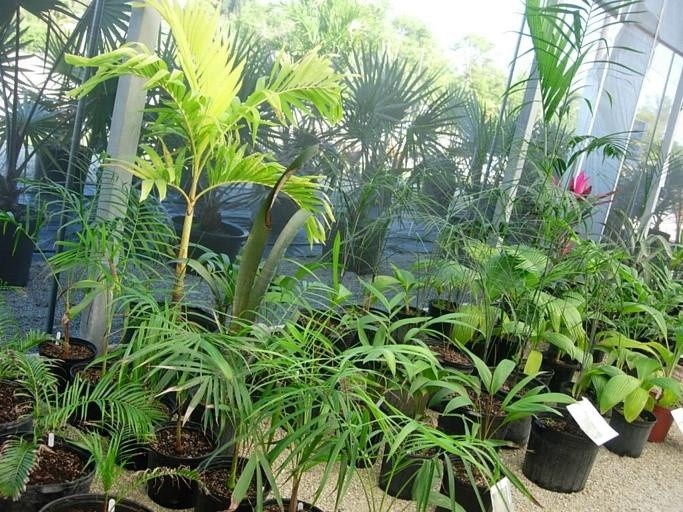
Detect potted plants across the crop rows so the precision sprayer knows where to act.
[0,2,683,512]
